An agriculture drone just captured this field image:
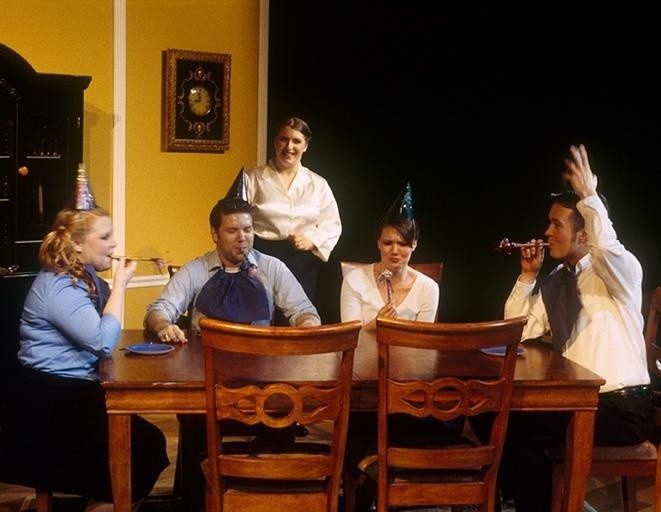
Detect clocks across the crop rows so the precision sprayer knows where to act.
[160,46,231,153]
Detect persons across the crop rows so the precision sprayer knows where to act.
[472,143,659,511]
[233,115,342,437]
[1,199,170,509]
[337,202,468,511]
[143,198,325,511]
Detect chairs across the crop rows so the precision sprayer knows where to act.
[357,316,528,512]
[544,289,661,512]
[333,261,448,324]
[195,316,363,512]
[165,263,187,497]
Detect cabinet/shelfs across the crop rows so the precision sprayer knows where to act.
[0,43,95,482]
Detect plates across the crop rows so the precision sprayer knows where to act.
[479,345,526,357]
[127,343,176,355]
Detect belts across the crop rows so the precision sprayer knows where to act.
[598,385,651,400]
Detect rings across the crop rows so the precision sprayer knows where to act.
[164,334,169,338]
